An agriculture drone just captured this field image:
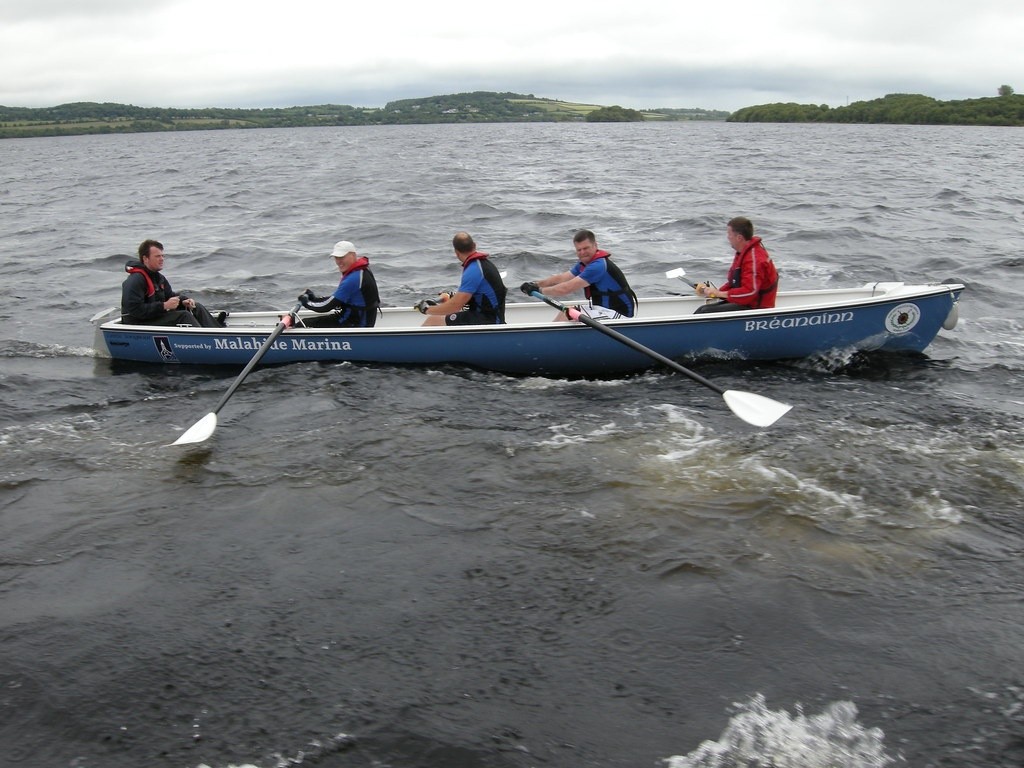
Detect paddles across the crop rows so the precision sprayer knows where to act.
[529,291,793,428]
[165,293,310,445]
[666,268,700,290]
[415,271,508,311]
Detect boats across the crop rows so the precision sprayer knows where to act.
[89,281,965,380]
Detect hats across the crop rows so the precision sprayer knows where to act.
[329,241,357,258]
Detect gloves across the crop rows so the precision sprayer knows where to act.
[520,281,542,296]
[305,289,316,302]
[298,295,307,306]
[414,299,430,315]
[438,288,456,298]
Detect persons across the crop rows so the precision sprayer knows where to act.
[411,231,508,326]
[119,239,222,328]
[692,218,779,315]
[295,241,380,327]
[520,229,635,322]
